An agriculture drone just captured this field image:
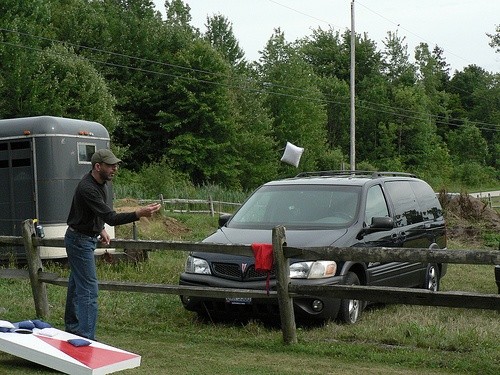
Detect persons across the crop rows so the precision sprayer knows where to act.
[64,148,162,341]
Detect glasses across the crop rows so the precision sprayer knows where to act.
[112,165,119,169]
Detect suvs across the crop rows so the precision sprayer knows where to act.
[177,169,448,328]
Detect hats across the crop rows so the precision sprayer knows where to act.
[92,149,121,164]
